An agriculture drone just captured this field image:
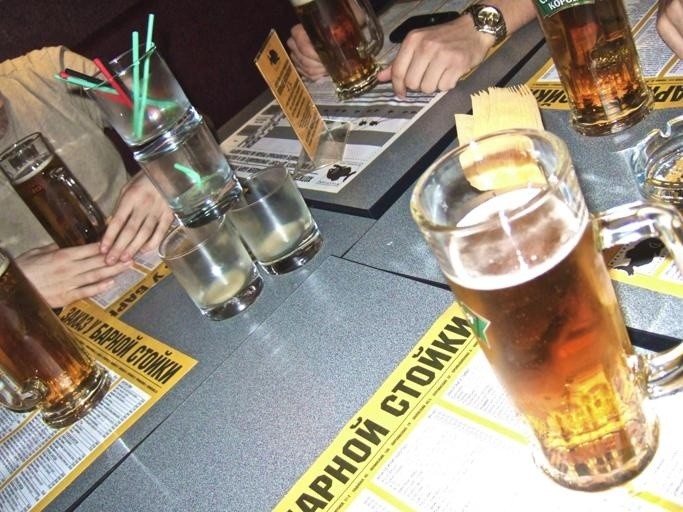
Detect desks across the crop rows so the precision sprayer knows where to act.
[343,0,683,343]
[214,1,545,217]
[72,257,682,512]
[2,194,375,512]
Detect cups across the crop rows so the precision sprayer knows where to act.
[409,127,683,492]
[0,248,112,428]
[531,0,653,137]
[224,164,325,277]
[0,132,109,250]
[288,0,384,101]
[134,105,244,230]
[83,42,203,163]
[157,212,264,323]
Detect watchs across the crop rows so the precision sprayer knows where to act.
[466,4,507,46]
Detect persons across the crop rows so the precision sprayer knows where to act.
[655,0,683,60]
[0,45,176,310]
[287,0,535,98]
